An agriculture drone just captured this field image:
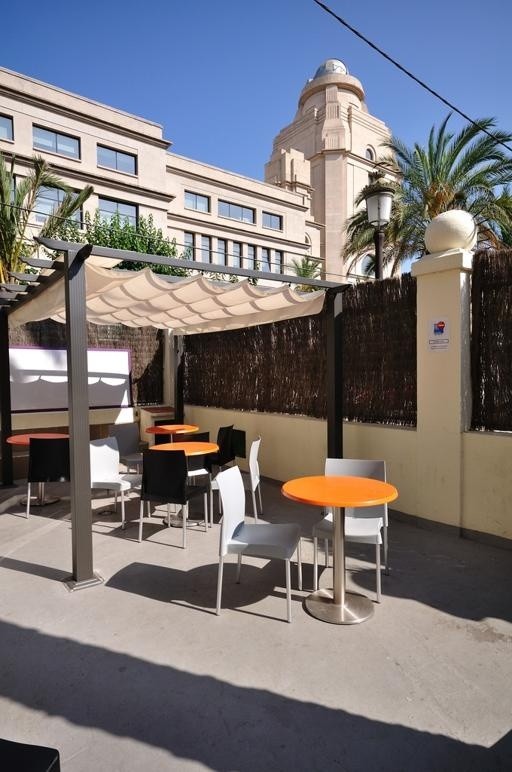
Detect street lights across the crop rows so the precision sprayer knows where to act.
[362,182,395,280]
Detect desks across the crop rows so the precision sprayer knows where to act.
[149,442,219,528]
[281,476,398,625]
[145,424,199,442]
[140,406,175,445]
[7,434,70,507]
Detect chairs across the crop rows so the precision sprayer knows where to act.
[211,435,263,524]
[26,438,70,518]
[89,437,150,530]
[209,425,245,472]
[154,420,175,444]
[108,422,143,474]
[213,466,303,623]
[173,432,212,485]
[313,457,389,604]
[138,449,208,548]
